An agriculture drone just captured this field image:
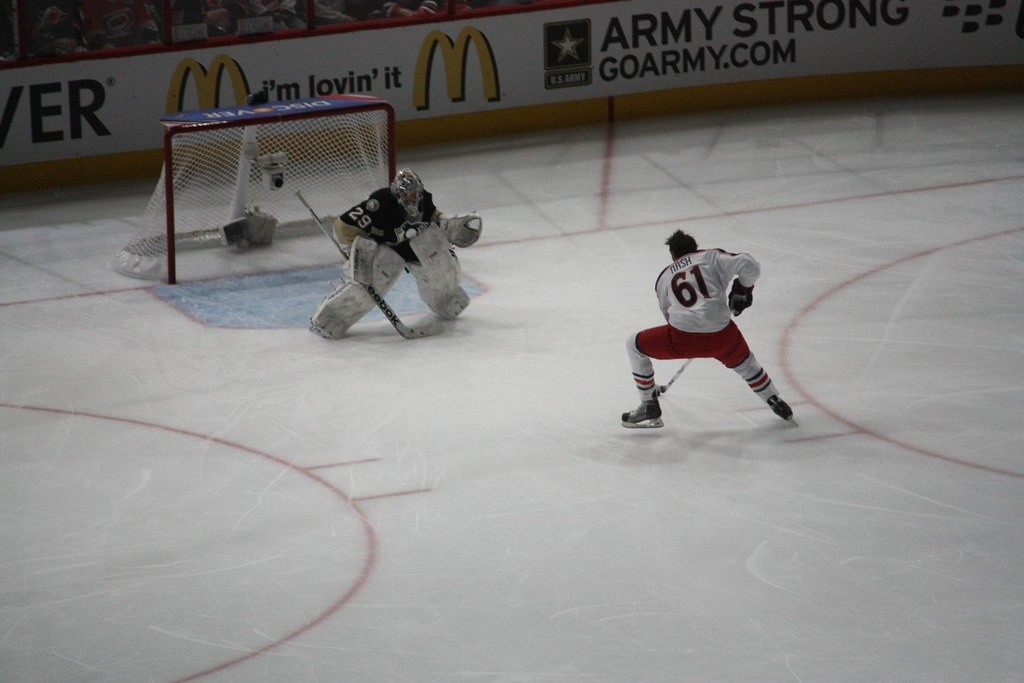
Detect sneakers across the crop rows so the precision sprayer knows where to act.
[621,389,664,428]
[767,394,799,426]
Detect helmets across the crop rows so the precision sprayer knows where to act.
[391,168,424,216]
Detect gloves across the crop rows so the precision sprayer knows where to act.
[728,278,754,316]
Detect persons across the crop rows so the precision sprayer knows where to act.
[621,230,798,428]
[309,168,482,341]
[0,0,526,69]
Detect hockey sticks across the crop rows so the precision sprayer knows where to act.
[655,297,743,395]
[294,187,446,340]
